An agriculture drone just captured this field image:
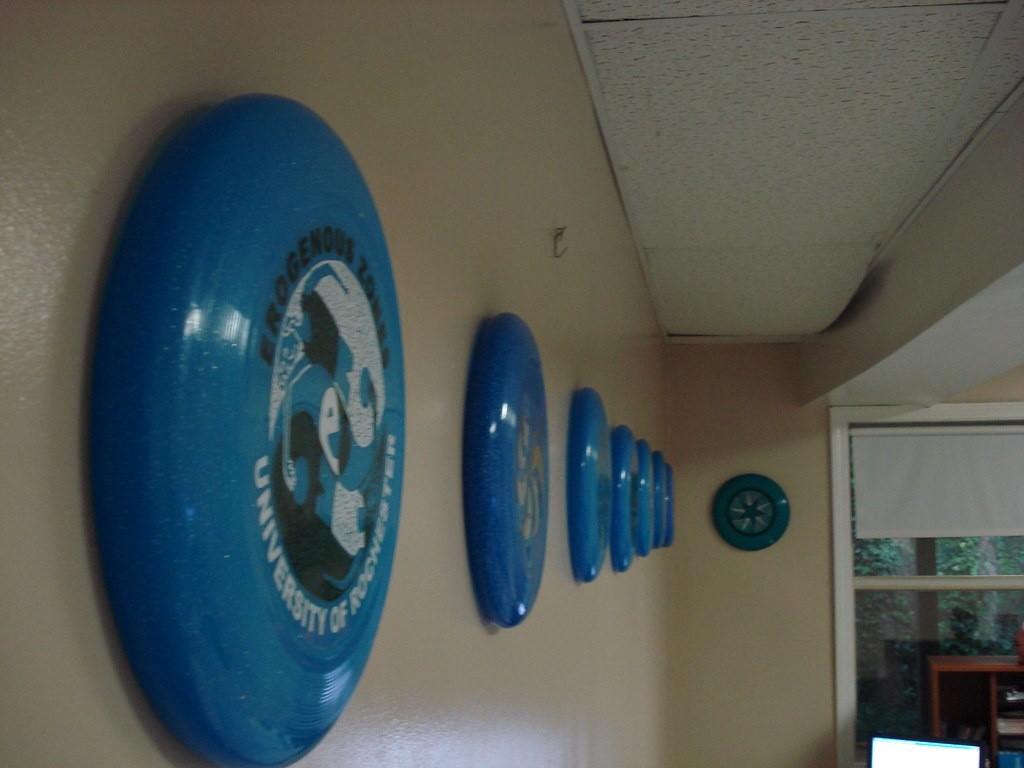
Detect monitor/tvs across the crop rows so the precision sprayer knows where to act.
[866,733,985,768]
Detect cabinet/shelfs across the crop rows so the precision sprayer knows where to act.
[927,655,1024,768]
